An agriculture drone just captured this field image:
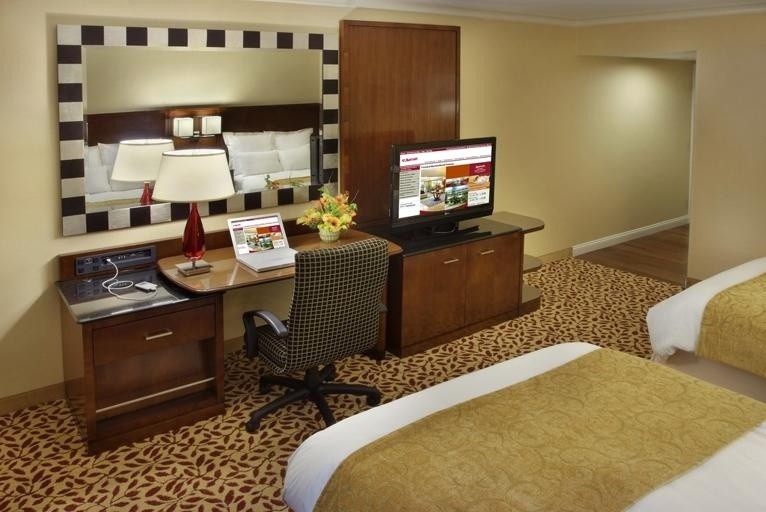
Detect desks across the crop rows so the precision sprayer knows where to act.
[156,229,401,293]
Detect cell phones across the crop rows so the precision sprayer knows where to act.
[134,281,157,292]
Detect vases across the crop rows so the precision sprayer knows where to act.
[320,226,341,244]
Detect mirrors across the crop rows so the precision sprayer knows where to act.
[55,24,340,238]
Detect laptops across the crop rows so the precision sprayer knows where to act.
[227,211,299,273]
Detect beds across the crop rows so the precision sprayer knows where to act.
[282,342,764,508]
[644,255,764,394]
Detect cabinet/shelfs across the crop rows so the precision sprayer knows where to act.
[364,211,545,361]
[56,266,226,454]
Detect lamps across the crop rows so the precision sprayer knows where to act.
[172,113,223,140]
[149,147,236,279]
[110,139,176,203]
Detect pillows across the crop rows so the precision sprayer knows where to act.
[225,128,313,175]
[85,140,153,193]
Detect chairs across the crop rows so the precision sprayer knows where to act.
[241,236,391,436]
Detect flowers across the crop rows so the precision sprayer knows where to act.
[297,175,360,234]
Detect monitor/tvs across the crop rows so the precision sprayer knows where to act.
[389,136,497,241]
